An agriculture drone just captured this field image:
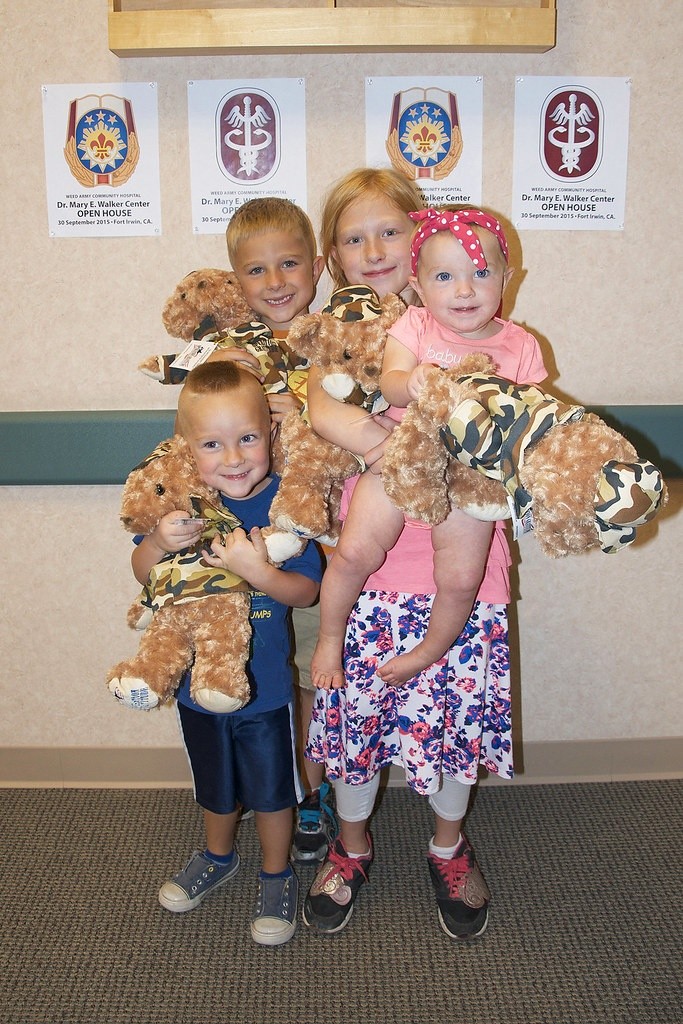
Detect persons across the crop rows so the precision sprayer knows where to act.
[303,169,561,940]
[134,362,326,941]
[310,205,549,690]
[197,195,338,863]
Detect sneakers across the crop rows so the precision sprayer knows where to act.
[427,830,490,942]
[289,782,335,866]
[158,846,241,913]
[301,829,374,934]
[249,863,299,945]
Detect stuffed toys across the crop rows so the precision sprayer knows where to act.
[140,268,343,548]
[109,434,305,713]
[388,352,669,556]
[269,285,408,538]
